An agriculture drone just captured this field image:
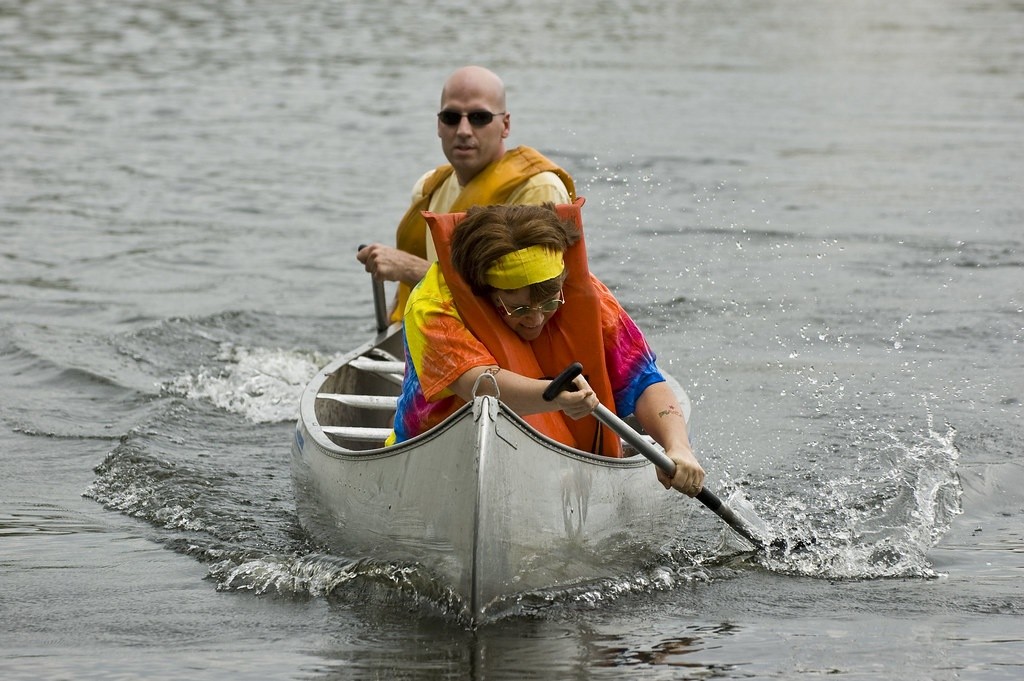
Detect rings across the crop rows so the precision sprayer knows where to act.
[692,483,701,489]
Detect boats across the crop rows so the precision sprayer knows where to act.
[292,320,691,622]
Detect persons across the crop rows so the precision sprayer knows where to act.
[358,67,578,323]
[384,201,706,497]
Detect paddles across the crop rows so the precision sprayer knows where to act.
[541,360,774,549]
[359,244,391,336]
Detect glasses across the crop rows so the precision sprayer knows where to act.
[437,111,505,127]
[494,287,565,319]
